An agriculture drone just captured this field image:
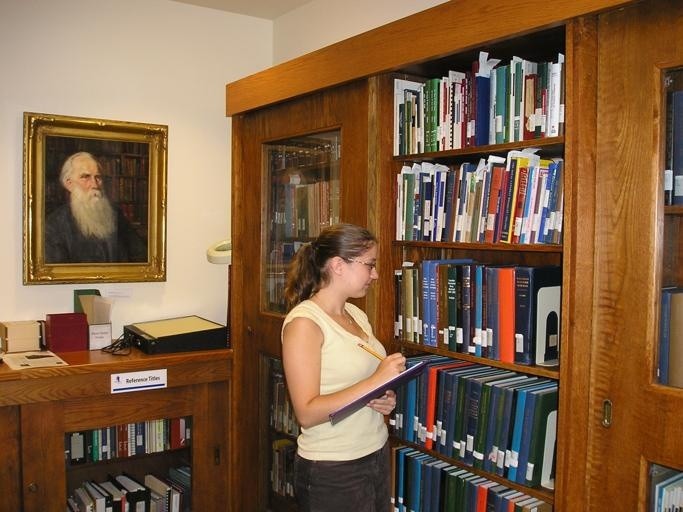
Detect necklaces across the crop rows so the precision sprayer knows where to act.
[333,313,354,324]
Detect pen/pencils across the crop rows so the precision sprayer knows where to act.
[358,343,384,361]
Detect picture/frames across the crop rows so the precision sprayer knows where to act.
[23,111,167,286]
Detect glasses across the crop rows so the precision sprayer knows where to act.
[329,256,376,270]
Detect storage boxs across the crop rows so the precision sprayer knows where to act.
[0,320,41,354]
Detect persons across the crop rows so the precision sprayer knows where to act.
[282,221,409,512]
[45,150,149,263]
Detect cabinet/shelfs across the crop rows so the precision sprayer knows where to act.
[584,0,683,511]
[368,0,597,512]
[226,0,367,511]
[0,339,234,512]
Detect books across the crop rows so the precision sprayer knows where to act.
[393,259,562,365]
[658,105,683,388]
[393,445,552,512]
[648,464,683,511]
[392,354,558,488]
[66,417,192,512]
[391,51,566,157]
[268,381,301,506]
[329,359,431,425]
[262,133,342,314]
[396,148,565,244]
